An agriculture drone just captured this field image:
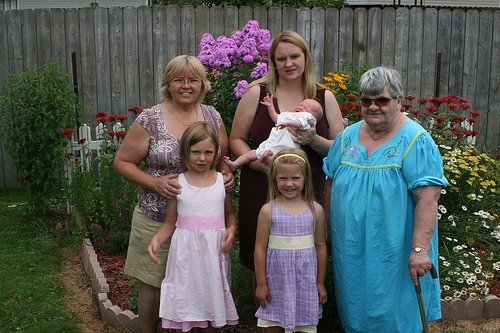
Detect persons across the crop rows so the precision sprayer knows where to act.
[113,54,235,333]
[223,93,323,171]
[323,66,449,333]
[229,31,345,309]
[147,121,240,333]
[255,147,327,333]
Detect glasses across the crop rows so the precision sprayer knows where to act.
[171,77,200,86]
[361,96,393,106]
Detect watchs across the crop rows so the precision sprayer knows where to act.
[411,247,428,254]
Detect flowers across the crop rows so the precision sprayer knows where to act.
[61,20,500,302]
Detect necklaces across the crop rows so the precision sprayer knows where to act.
[171,98,199,126]
